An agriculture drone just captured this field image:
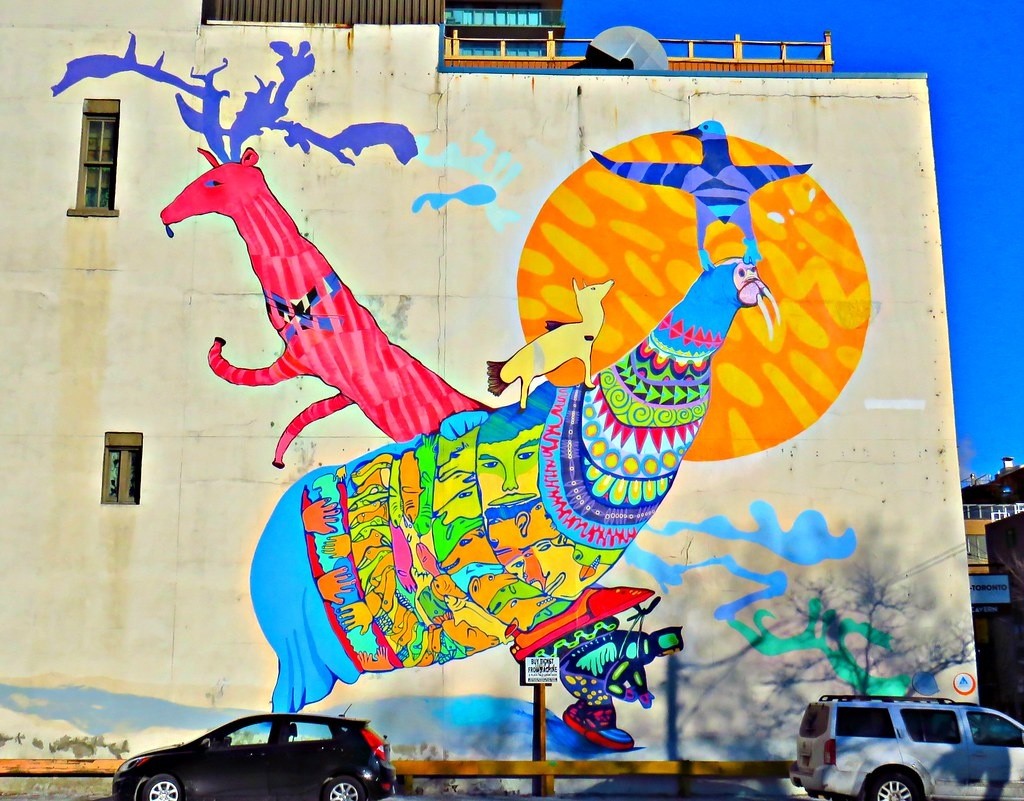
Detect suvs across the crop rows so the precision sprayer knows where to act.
[788,695,1024,801]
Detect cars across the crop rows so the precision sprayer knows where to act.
[111,712,396,801]
